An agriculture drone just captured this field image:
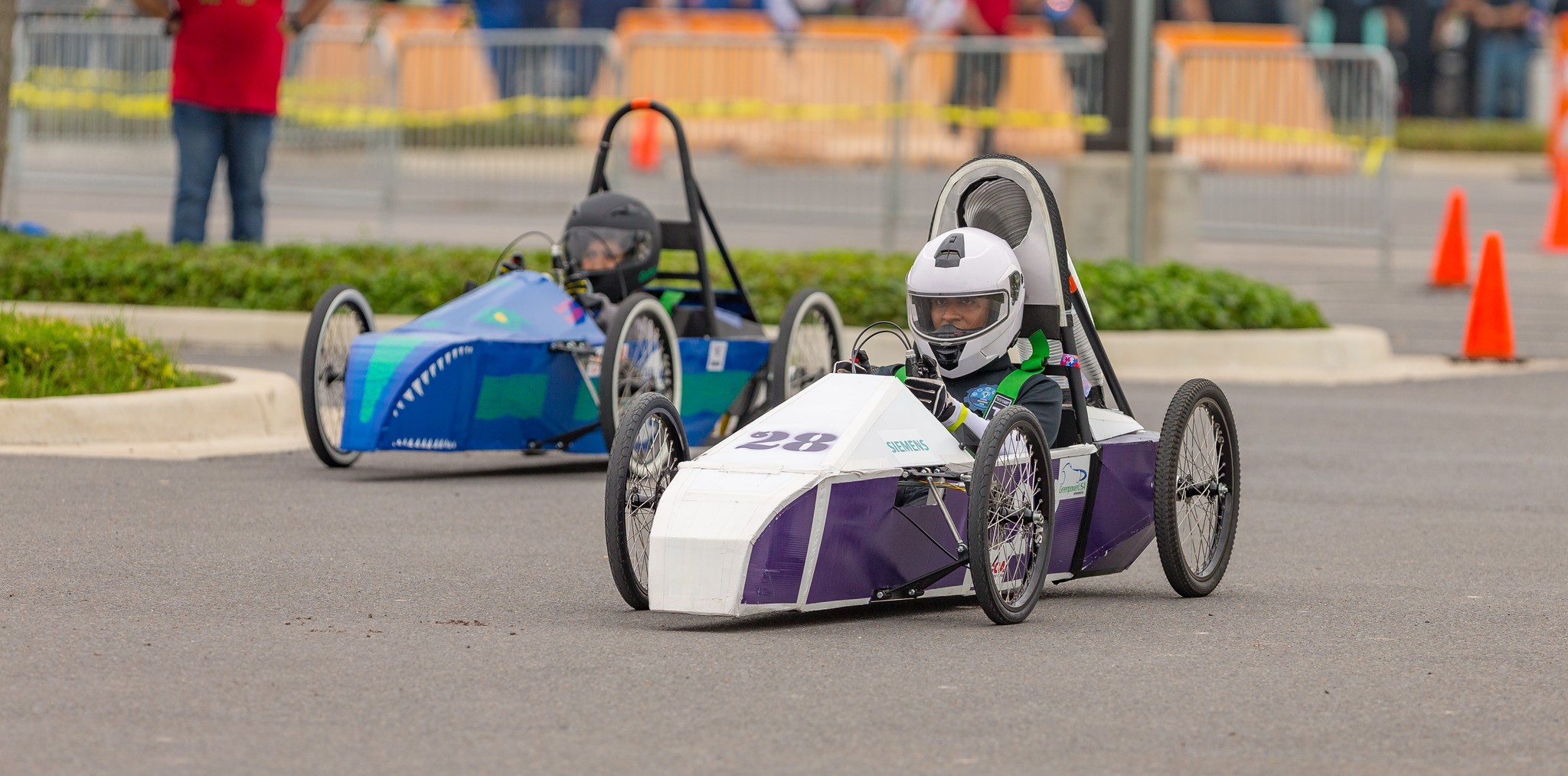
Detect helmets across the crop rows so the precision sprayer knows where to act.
[904,227,1025,379]
[558,191,663,308]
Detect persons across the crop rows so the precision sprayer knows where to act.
[942,0,1568,172]
[852,228,1063,458]
[497,193,662,343]
[131,0,330,247]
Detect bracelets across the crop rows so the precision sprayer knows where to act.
[287,18,302,32]
[163,12,181,37]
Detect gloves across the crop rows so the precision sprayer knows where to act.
[904,354,955,422]
[564,272,594,301]
[834,349,872,374]
[496,252,525,276]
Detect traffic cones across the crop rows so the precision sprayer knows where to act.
[1425,191,1471,296]
[1445,234,1527,365]
[628,111,660,176]
[1540,188,1568,254]
